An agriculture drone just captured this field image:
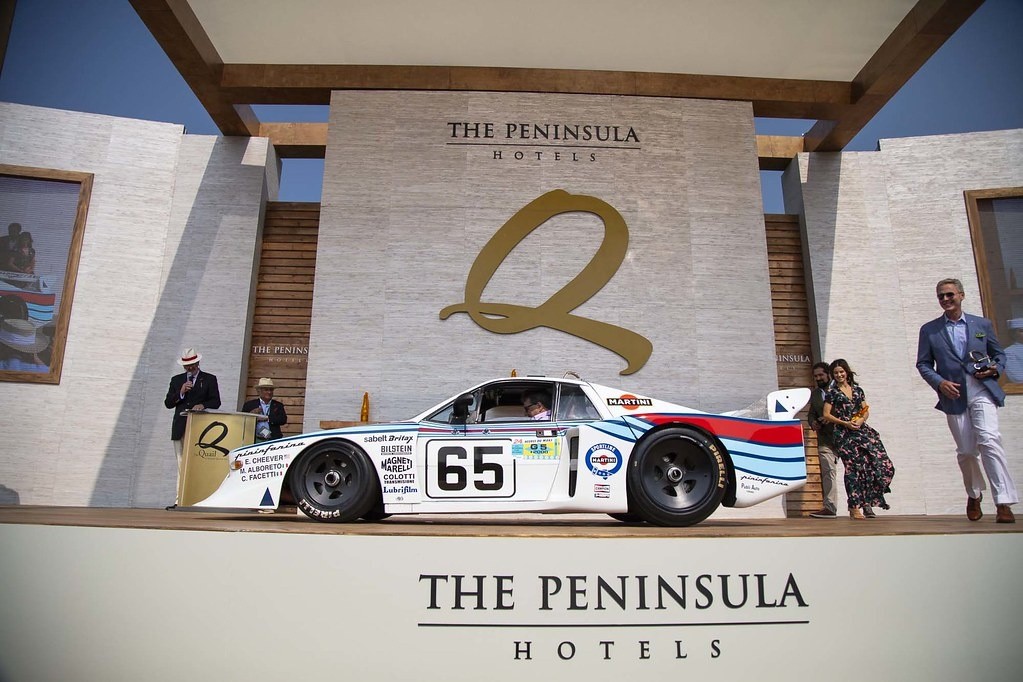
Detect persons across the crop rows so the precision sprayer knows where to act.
[915,279,1019,522]
[0,223,36,274]
[241,378,287,444]
[807,362,875,518]
[524,394,558,420]
[165,348,221,509]
[823,359,896,519]
[1003,318,1023,383]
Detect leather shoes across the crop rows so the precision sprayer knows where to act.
[996,505,1016,523]
[967,492,982,520]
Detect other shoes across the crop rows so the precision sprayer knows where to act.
[850,509,864,520]
[166,504,177,511]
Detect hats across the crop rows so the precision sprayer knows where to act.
[253,378,279,387]
[1006,318,1023,330]
[177,348,203,365]
[0,319,49,353]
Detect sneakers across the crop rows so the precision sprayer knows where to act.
[863,507,876,517]
[809,509,837,518]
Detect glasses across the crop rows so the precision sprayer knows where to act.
[524,403,537,413]
[937,292,963,299]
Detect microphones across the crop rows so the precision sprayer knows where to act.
[187,372,193,392]
